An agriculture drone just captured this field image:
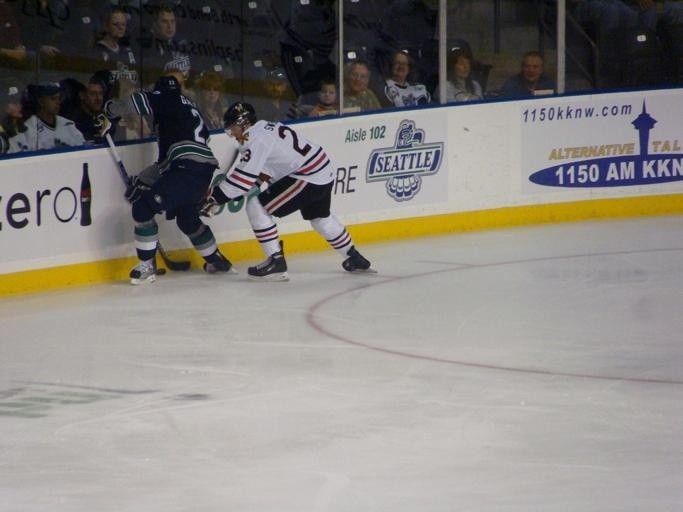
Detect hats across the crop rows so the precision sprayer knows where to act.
[1,85,24,103]
[35,80,65,97]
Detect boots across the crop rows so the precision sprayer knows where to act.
[130,257,157,279]
[203,249,232,274]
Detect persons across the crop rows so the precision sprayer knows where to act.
[1,0,683,155]
[92,75,231,282]
[200,101,369,276]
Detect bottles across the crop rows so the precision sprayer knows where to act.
[79,161,94,227]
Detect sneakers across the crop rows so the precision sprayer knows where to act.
[342,245,370,271]
[248,241,287,276]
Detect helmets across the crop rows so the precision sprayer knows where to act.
[153,76,181,92]
[224,102,254,128]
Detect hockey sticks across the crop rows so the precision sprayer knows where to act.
[105,131,191,270]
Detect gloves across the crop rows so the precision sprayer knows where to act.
[124,176,152,201]
[201,186,217,218]
[92,100,120,145]
